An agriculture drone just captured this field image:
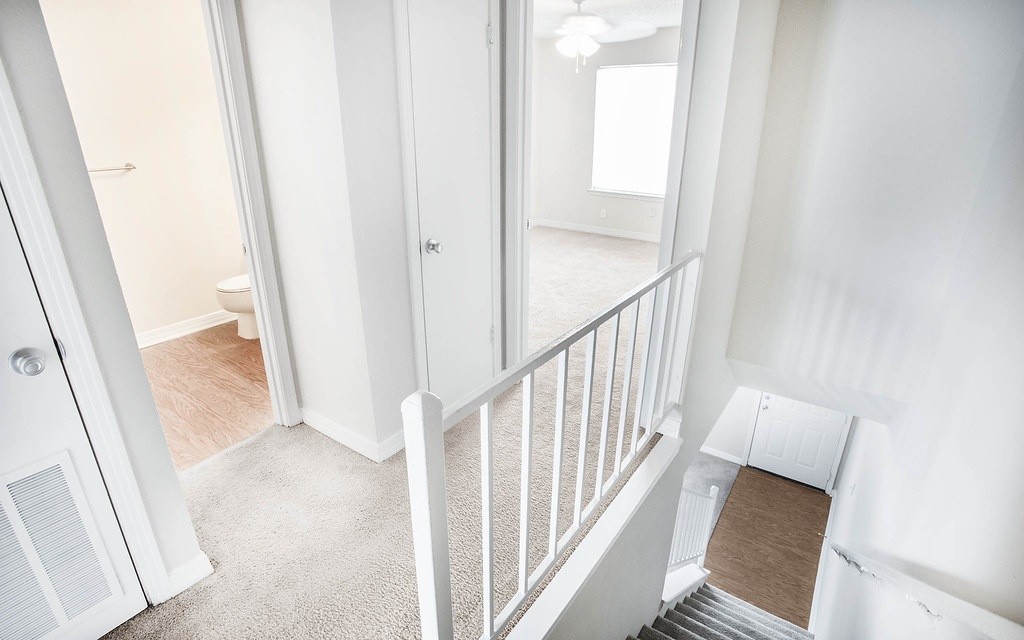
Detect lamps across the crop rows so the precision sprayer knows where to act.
[553,0,613,73]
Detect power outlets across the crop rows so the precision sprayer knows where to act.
[600,208,607,218]
[648,206,656,217]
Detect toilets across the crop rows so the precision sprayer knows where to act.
[215,273,260,339]
